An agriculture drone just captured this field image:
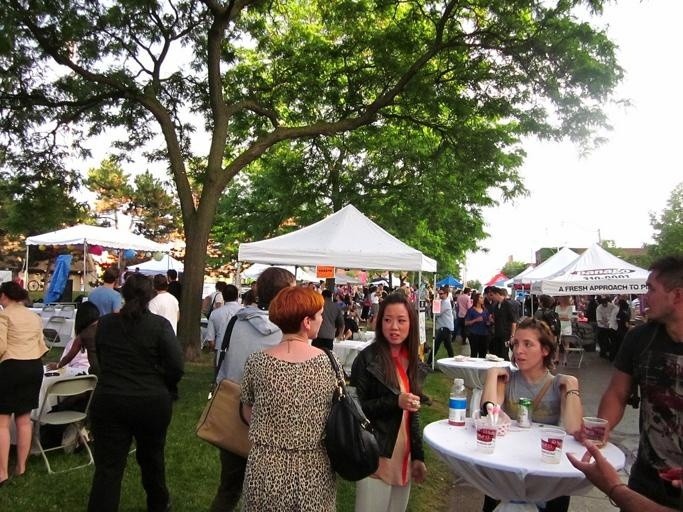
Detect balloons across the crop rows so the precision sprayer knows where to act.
[38,245,46,251]
[153,252,165,261]
[123,250,135,259]
[146,252,152,258]
[89,245,102,256]
[140,251,144,258]
[52,244,59,249]
[66,244,77,251]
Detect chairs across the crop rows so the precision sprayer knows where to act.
[60,305,75,319]
[42,316,70,353]
[560,335,586,370]
[42,306,55,316]
[28,374,98,475]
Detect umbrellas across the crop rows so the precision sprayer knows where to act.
[24,224,169,296]
[530,243,651,318]
[484,273,510,288]
[514,247,581,317]
[236,203,437,372]
[503,266,534,316]
[239,261,356,284]
[436,276,463,292]
[127,253,184,278]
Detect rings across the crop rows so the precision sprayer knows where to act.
[412,399,419,408]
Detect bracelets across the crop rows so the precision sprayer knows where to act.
[609,484,628,507]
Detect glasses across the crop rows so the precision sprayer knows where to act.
[439,292,446,295]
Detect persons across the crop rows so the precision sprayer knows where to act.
[87,274,183,511]
[465,293,492,357]
[203,281,226,320]
[580,295,599,329]
[124,267,133,280]
[206,286,243,374]
[617,300,631,348]
[383,283,419,305]
[526,295,532,316]
[630,294,648,326]
[481,319,582,512]
[535,294,561,354]
[576,256,683,512]
[552,294,573,365]
[351,295,428,512]
[596,295,617,361]
[489,287,516,360]
[47,302,102,376]
[484,286,495,314]
[452,288,472,346]
[236,288,345,512]
[166,269,180,301]
[90,269,121,316]
[135,268,140,273]
[360,288,372,324]
[207,266,296,512]
[419,285,431,316]
[470,290,478,301]
[250,282,262,303]
[483,286,494,311]
[532,294,538,315]
[499,288,523,320]
[146,274,180,336]
[0,282,49,487]
[566,439,683,512]
[300,280,362,348]
[428,287,454,366]
[517,293,523,317]
[370,287,379,330]
[452,297,459,343]
[378,284,389,304]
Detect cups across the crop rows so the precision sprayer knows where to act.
[539,428,565,466]
[579,415,609,450]
[474,417,495,451]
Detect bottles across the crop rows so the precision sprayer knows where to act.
[518,397,533,428]
[449,378,467,427]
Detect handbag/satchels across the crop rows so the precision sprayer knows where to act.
[206,293,221,320]
[114,312,178,400]
[195,316,253,460]
[325,347,380,481]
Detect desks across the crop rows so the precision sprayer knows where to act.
[26,308,77,320]
[332,339,368,376]
[10,364,92,445]
[422,419,626,512]
[436,357,511,419]
[353,331,375,341]
[40,318,75,348]
